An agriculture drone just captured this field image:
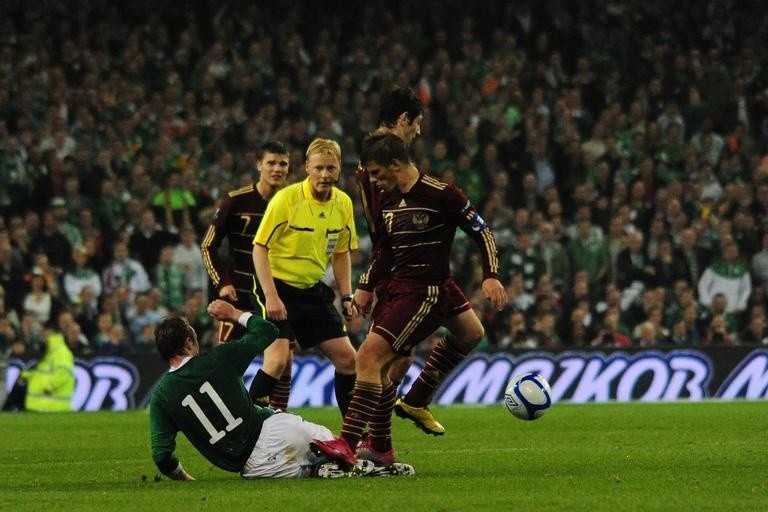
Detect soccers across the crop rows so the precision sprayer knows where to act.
[504,373,551,420]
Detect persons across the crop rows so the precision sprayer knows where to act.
[251,137,358,421]
[21,334,76,413]
[148,299,417,480]
[1,0,768,363]
[356,84,486,438]
[199,139,294,413]
[308,132,510,471]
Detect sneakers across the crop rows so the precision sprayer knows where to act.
[393,398,445,436]
[308,435,415,479]
